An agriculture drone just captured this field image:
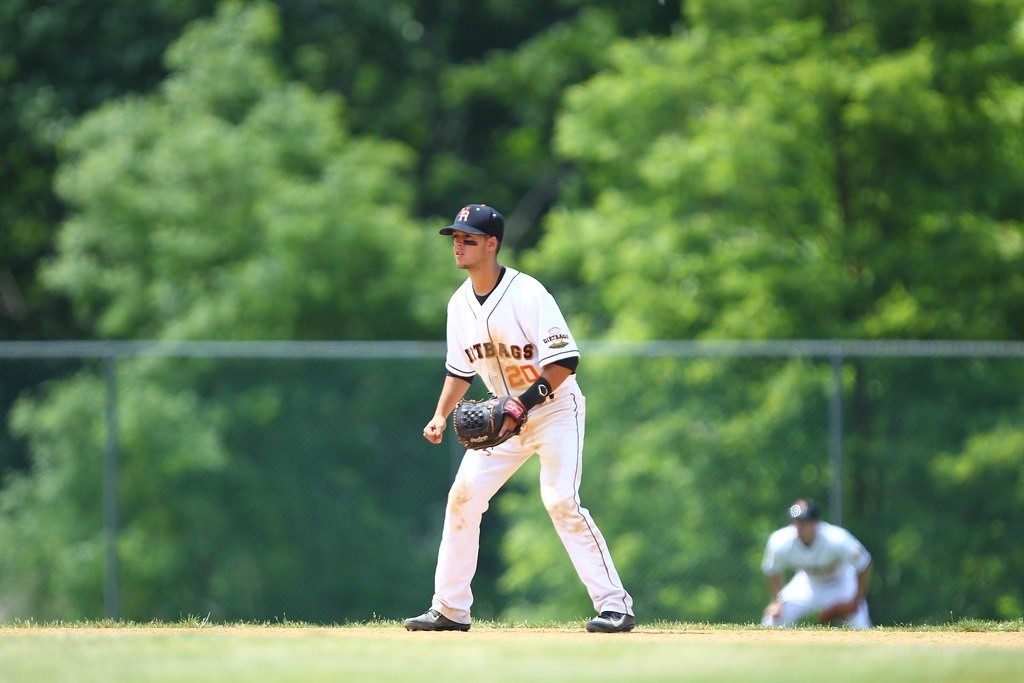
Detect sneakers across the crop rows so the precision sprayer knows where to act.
[586,611,636,633]
[403,609,471,632]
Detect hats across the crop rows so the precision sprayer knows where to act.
[790,495,816,522]
[439,202,506,239]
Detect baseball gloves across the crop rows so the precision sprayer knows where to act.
[452,395,529,450]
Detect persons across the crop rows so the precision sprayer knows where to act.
[404,203,635,632]
[760,498,872,628]
